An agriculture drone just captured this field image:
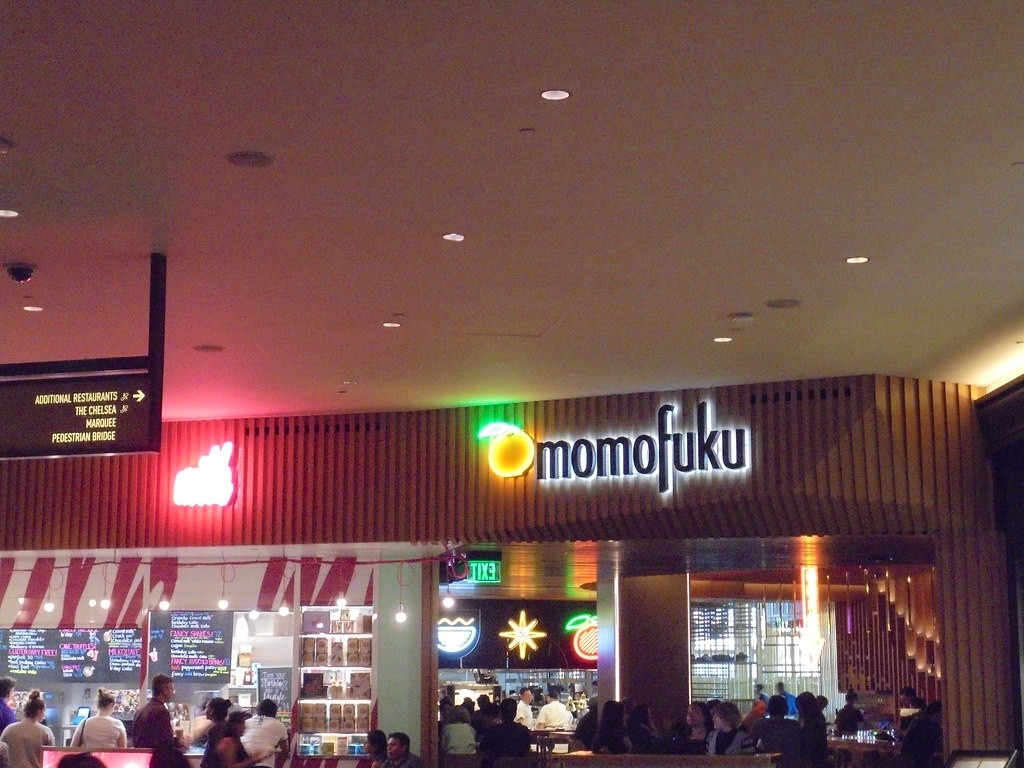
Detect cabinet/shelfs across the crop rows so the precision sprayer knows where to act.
[298,606,373,759]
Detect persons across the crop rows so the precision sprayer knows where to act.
[441,705,476,754]
[0,691,55,768]
[215,712,275,768]
[480,699,531,754]
[381,732,421,768]
[132,674,176,748]
[439,685,598,730]
[835,686,942,768]
[575,682,828,754]
[70,689,127,749]
[240,698,288,768]
[0,678,16,735]
[200,697,228,768]
[363,729,388,768]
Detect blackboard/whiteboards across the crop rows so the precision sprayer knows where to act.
[0,626,142,683]
[145,609,233,683]
[257,667,292,713]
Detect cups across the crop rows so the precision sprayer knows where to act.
[149,652,158,662]
[857,730,873,737]
